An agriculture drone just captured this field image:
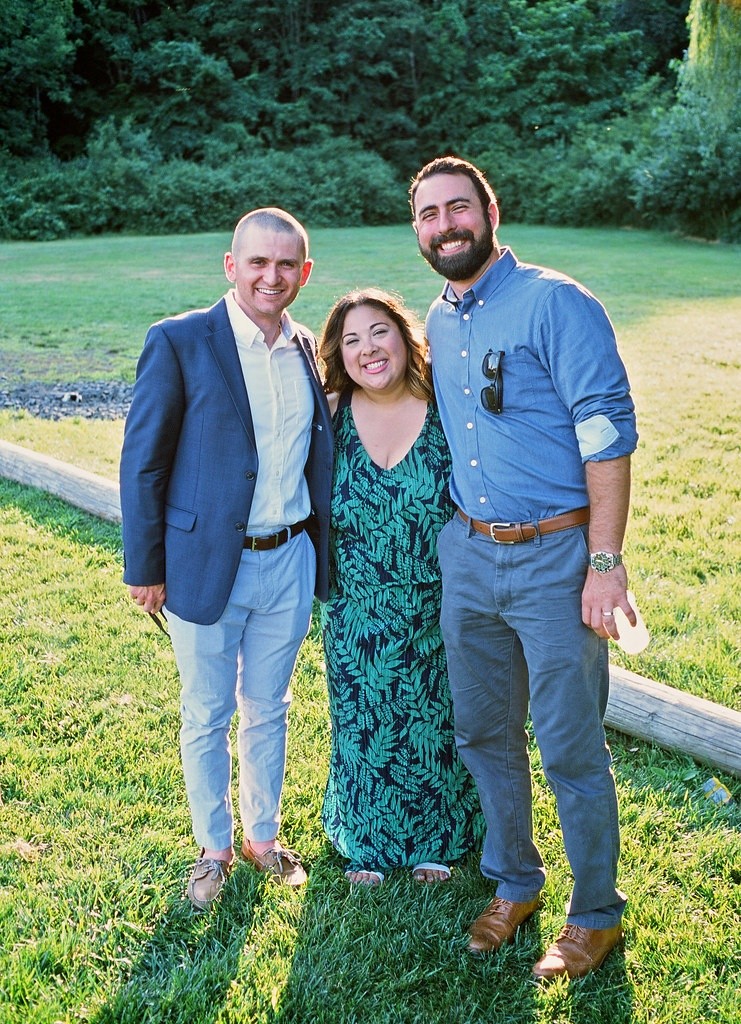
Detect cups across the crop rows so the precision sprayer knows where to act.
[602,590,650,655]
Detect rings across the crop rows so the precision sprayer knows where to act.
[601,611,613,616]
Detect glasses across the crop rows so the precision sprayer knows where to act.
[148,607,172,644]
[479,349,506,416]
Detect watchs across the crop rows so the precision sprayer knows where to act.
[586,551,623,573]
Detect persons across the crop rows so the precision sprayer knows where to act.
[116,205,333,912]
[408,156,637,982]
[314,286,487,891]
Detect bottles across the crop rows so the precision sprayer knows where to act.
[703,778,732,808]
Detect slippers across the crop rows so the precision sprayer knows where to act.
[344,866,385,889]
[412,862,452,883]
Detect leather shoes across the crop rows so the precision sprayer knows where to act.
[463,894,540,957]
[530,920,625,981]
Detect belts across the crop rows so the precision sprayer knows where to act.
[239,511,316,550]
[457,505,591,545]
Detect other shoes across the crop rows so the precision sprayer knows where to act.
[186,846,236,907]
[241,839,309,889]
